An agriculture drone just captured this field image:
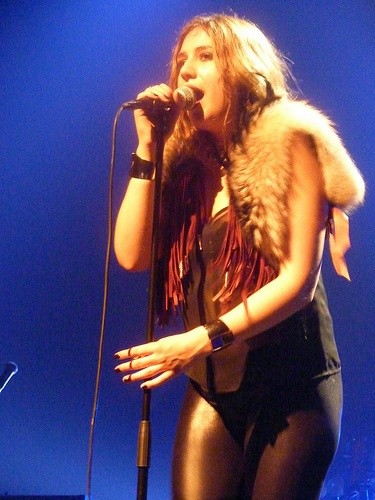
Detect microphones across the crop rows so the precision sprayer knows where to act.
[122,85,195,111]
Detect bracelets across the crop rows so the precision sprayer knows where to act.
[128,151,155,182]
[203,319,224,352]
[216,320,234,345]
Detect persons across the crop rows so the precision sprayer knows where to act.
[111,12,367,500]
